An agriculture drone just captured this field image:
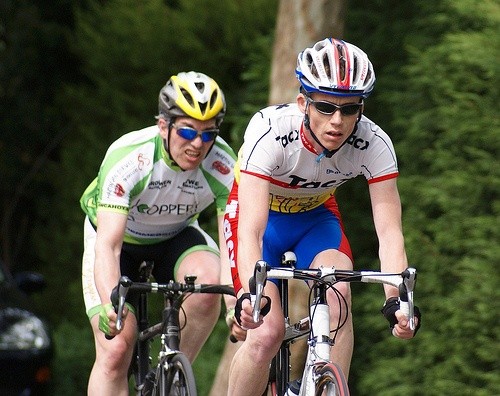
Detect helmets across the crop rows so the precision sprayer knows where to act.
[159,72,227,122]
[295,38,375,96]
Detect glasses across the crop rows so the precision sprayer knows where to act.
[172,123,220,143]
[305,96,363,116]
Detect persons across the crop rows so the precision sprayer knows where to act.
[225,37,421,396]
[80,71,238,396]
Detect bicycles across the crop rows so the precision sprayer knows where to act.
[103,260,238,396]
[242,251,418,396]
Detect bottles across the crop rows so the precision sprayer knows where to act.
[284,380,301,396]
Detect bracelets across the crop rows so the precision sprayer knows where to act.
[383,297,398,306]
[225,307,235,316]
